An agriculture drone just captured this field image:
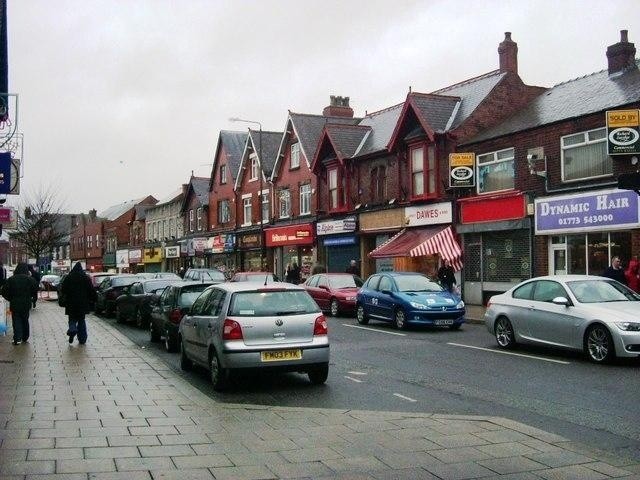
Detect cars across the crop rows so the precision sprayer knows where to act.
[229,271,281,282]
[483,273,640,365]
[137,272,180,280]
[115,278,181,329]
[297,272,364,317]
[38,271,144,317]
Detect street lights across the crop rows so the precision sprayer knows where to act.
[228,117,267,273]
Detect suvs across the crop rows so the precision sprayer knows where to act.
[356,272,466,330]
[177,270,330,391]
[184,269,228,282]
[149,281,222,352]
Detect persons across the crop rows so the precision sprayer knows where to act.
[1,262,37,346]
[287,264,301,287]
[624,256,640,294]
[437,258,456,292]
[604,257,628,286]
[57,262,98,345]
[27,264,41,311]
